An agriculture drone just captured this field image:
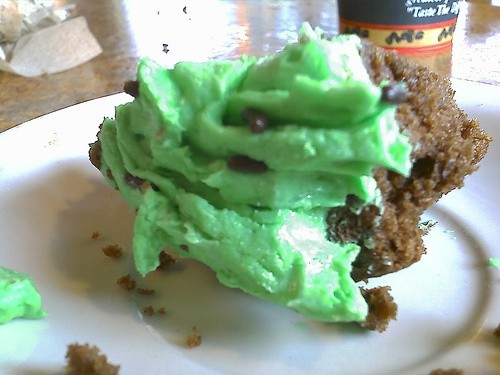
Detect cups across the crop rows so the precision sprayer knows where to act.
[338,0,459,54]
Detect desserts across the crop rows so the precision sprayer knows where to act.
[87,23,494,332]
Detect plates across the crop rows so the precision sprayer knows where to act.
[0,91,499,374]
